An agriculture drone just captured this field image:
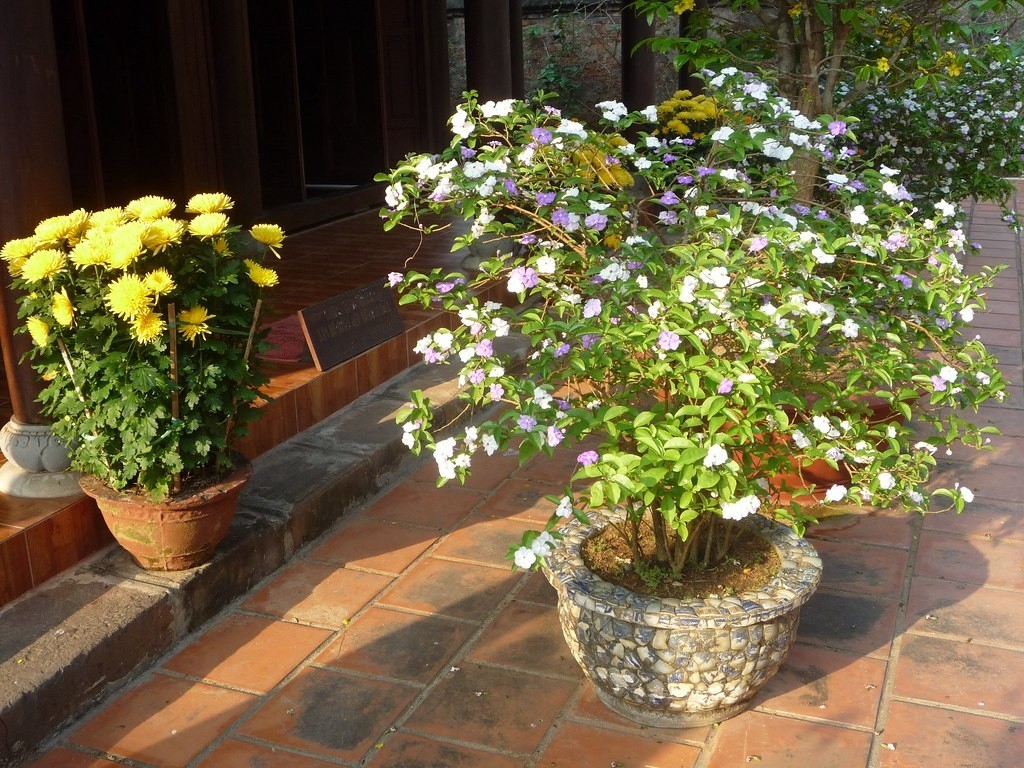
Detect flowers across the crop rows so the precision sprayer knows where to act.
[371,1,1023,573]
[0,193,288,491]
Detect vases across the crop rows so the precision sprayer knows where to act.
[538,507,824,730]
[717,382,922,516]
[79,453,252,570]
[784,350,929,429]
